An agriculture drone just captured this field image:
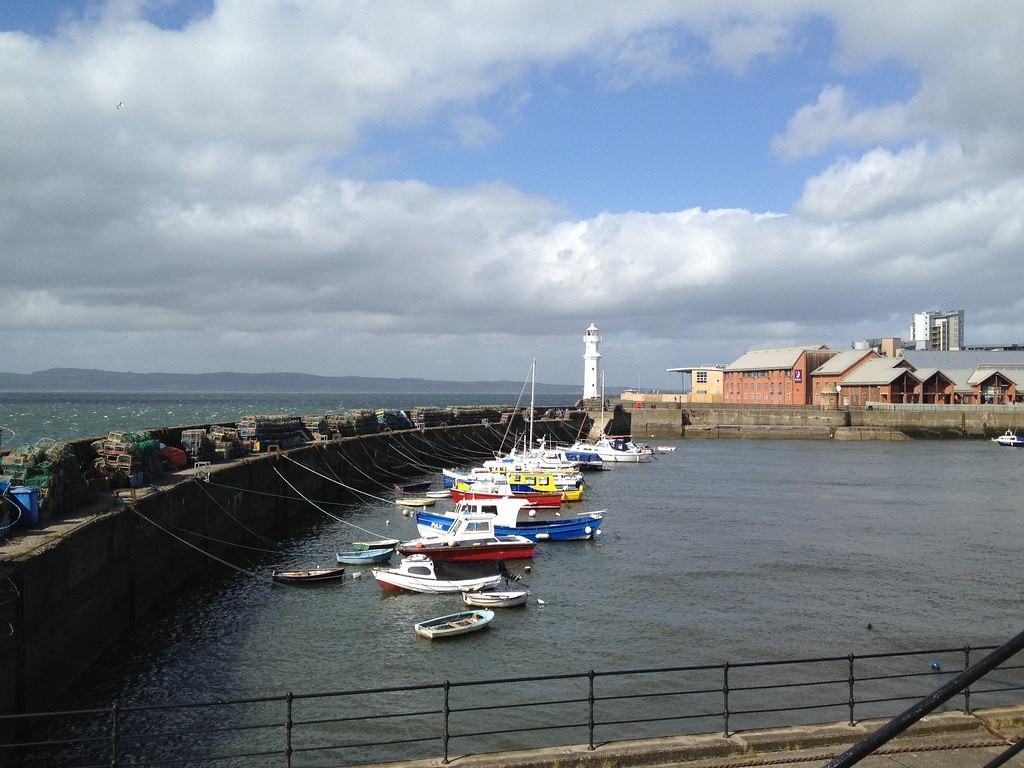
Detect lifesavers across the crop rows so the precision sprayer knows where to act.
[512,486,516,490]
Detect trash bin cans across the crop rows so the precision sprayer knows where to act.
[0,482,12,539]
[10,486,40,527]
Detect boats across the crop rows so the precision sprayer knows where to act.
[372,554,502,594]
[415,499,608,541]
[992,428,1024,447]
[462,591,529,608]
[396,498,436,505]
[337,548,393,565]
[426,491,450,497]
[442,357,676,503]
[272,567,345,584]
[415,610,495,640]
[394,509,538,560]
[352,539,399,551]
[450,478,563,508]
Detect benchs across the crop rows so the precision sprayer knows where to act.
[466,620,474,624]
[448,622,460,628]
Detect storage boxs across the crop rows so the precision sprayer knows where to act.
[122,471,144,488]
[0,480,12,498]
